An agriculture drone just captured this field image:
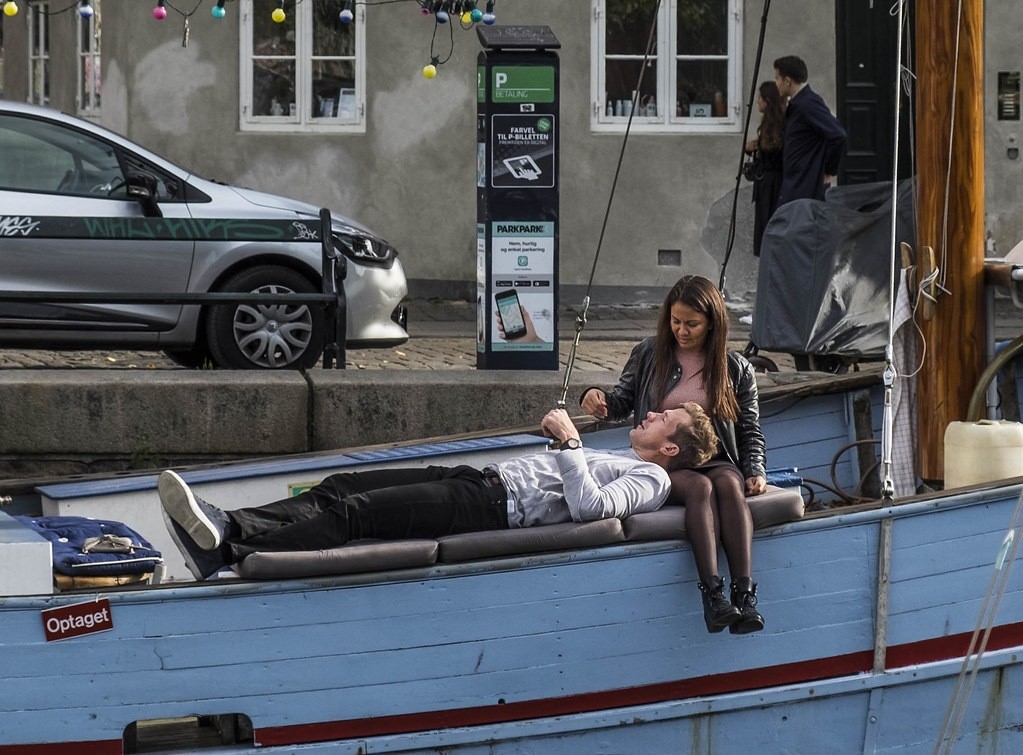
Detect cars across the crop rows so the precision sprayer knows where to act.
[0,101,409,370]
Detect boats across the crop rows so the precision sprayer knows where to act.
[0,337,1023,755]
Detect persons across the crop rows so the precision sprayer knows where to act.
[156,403,719,582]
[736,55,850,326]
[496,305,546,342]
[579,273,769,636]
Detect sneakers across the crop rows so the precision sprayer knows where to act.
[159,502,235,581]
[157,470,233,550]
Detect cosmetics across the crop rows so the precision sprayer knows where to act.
[606,90,657,117]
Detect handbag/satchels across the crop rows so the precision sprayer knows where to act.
[743,150,756,182]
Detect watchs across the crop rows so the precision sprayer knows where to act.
[560,437,583,451]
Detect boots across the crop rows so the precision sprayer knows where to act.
[727,576,764,634]
[698,576,741,633]
